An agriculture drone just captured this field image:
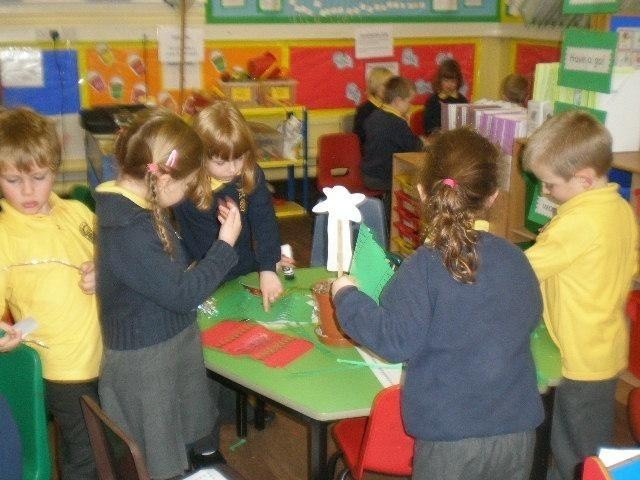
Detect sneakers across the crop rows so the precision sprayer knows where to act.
[252,409,275,424]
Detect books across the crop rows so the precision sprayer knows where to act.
[440,96,529,193]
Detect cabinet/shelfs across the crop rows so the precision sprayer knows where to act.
[508,137,640,408]
[389,151,508,261]
[83,127,122,205]
[233,105,312,222]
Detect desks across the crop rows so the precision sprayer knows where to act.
[191,268,426,480]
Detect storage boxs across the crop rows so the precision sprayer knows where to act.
[214,76,258,109]
[254,76,300,108]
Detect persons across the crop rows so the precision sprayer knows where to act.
[421,59,467,138]
[522,108,640,480]
[356,76,425,193]
[0,102,136,479]
[331,128,544,479]
[353,65,396,139]
[171,98,285,429]
[500,73,529,104]
[93,114,240,480]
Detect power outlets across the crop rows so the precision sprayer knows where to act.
[47,29,63,44]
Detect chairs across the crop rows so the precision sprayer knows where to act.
[79,393,250,479]
[0,329,51,480]
[315,133,386,199]
[310,196,387,267]
[408,107,431,137]
[326,384,416,479]
